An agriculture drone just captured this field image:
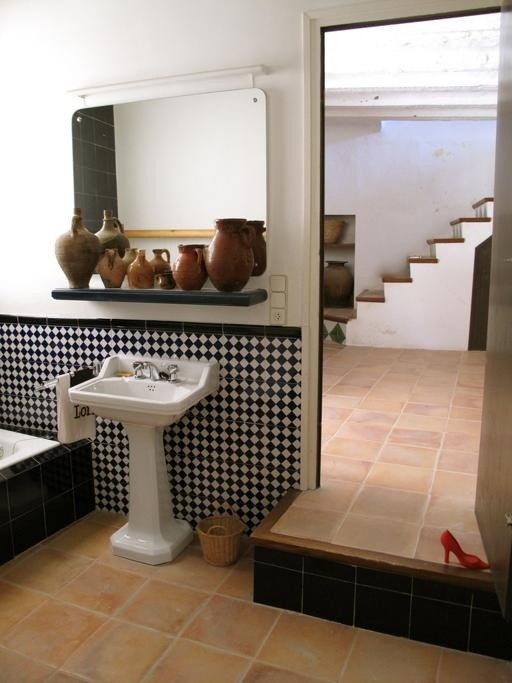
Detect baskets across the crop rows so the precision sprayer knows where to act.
[194,515,247,568]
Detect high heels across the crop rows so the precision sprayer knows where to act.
[439,528,491,570]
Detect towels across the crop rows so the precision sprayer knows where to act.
[57,367,97,442]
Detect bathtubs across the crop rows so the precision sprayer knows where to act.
[0,428,62,471]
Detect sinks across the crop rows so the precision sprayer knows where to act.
[68,354,220,430]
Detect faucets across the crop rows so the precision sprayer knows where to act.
[132,361,145,379]
[143,362,161,380]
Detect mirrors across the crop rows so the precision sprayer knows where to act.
[71,87,266,245]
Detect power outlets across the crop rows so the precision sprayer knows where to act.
[270,308,286,327]
